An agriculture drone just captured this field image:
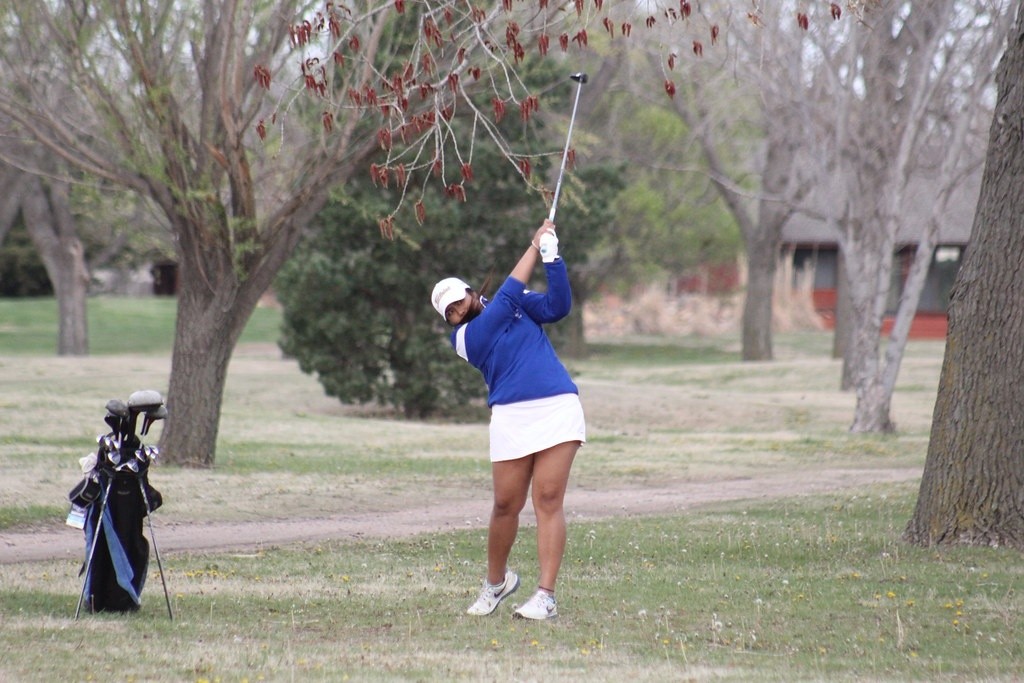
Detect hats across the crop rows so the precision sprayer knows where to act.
[436,280,474,326]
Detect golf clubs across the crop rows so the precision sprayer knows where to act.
[101,389,170,476]
[547,73,587,222]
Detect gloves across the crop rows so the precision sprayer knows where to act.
[533,221,565,260]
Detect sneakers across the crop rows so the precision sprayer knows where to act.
[519,578,556,627]
[470,572,523,619]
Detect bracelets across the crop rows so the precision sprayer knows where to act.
[531,240,539,251]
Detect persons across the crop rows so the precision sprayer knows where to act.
[431,218,586,621]
